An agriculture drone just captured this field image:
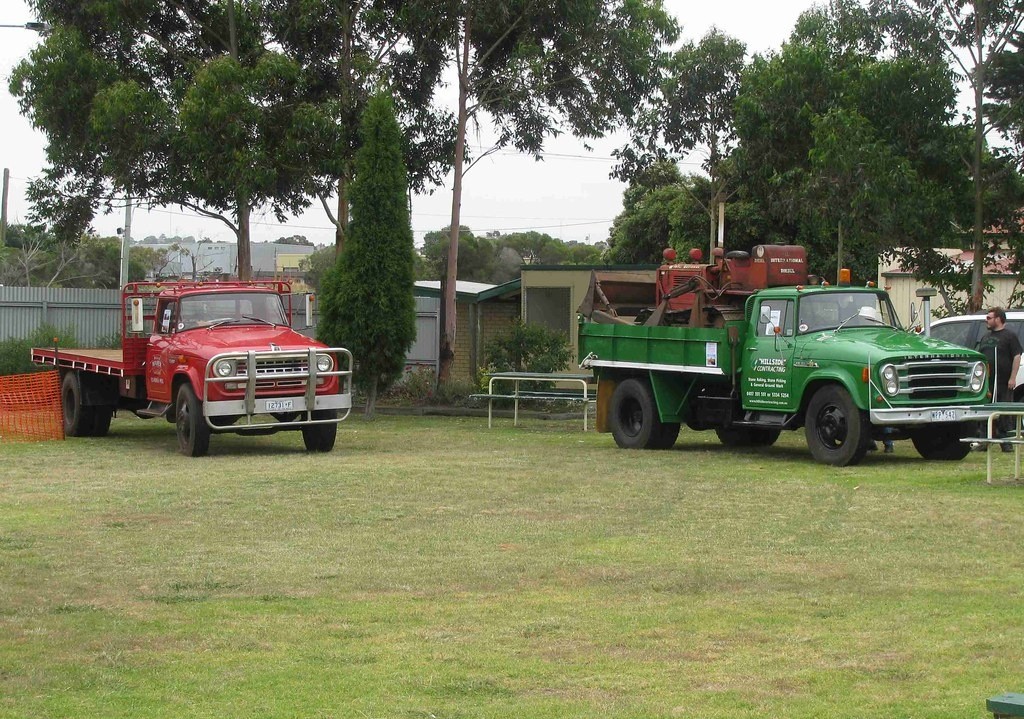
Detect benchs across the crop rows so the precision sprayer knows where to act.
[469,394,588,401]
[959,438,1024,444]
[512,390,596,398]
[1006,429,1024,437]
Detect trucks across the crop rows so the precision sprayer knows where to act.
[30,280,355,457]
[574,243,991,468]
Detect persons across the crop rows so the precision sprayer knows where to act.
[978,307,1023,453]
[868,440,893,453]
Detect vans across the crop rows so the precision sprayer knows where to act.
[909,311,1024,443]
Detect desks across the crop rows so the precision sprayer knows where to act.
[970,402,1024,484]
[485,371,596,431]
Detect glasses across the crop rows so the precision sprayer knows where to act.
[986,316,998,320]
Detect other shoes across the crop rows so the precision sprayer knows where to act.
[1002,444,1014,452]
[868,445,877,450]
[979,445,988,451]
[884,445,894,452]
